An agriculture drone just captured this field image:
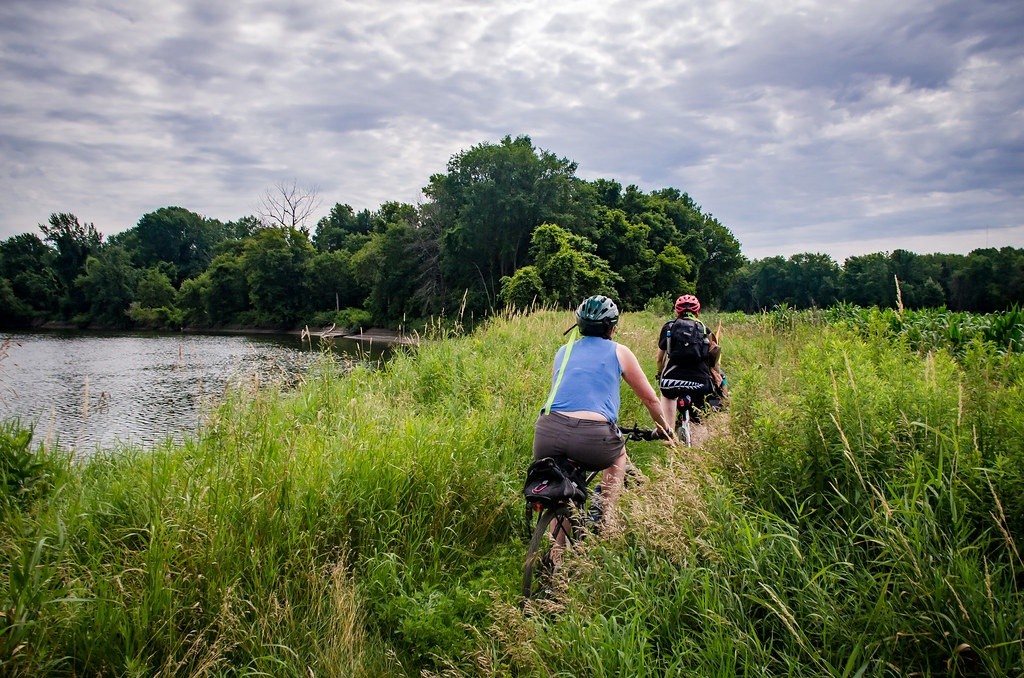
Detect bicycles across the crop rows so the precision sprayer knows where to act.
[519,422,671,616]
[655,370,726,451]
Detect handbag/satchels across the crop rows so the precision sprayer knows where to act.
[523,457,585,544]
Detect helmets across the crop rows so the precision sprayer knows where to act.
[675,294,701,318]
[575,296,619,336]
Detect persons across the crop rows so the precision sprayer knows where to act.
[533,295,679,568]
[655,294,722,431]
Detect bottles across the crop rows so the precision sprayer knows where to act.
[589,485,603,522]
